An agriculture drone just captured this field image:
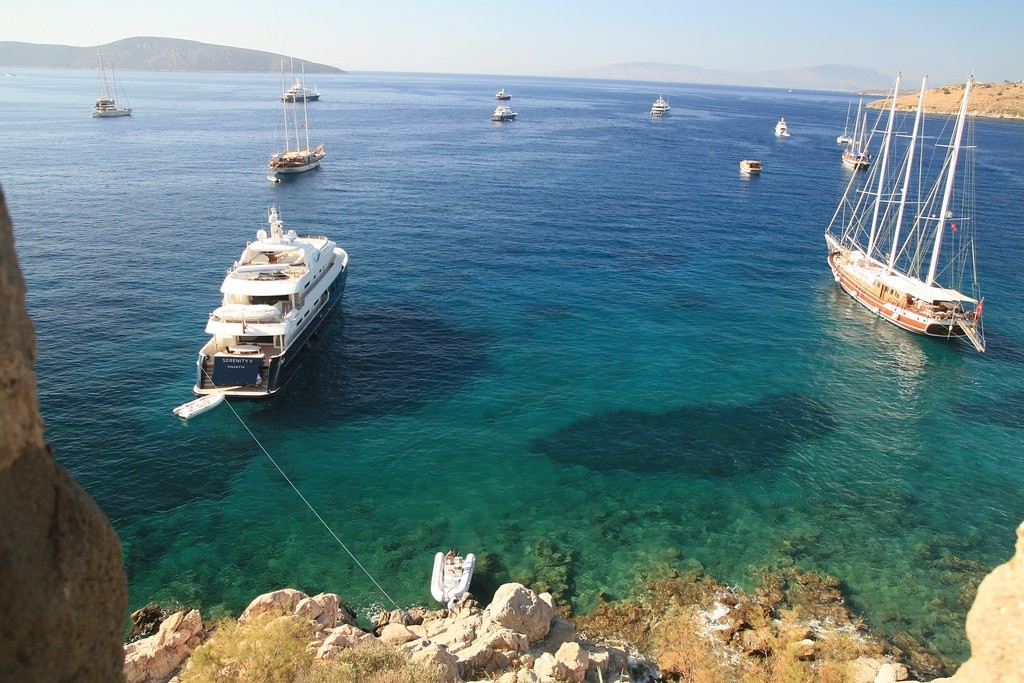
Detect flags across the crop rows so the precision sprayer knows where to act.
[974,299,984,323]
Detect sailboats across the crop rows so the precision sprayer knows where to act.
[841,97,871,169]
[268,56,325,173]
[837,97,852,144]
[92,40,135,117]
[823,71,987,353]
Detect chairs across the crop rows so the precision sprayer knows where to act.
[226,346,235,355]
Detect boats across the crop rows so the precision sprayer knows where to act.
[280,76,321,102]
[650,93,671,117]
[495,88,512,101]
[739,159,762,174]
[491,105,518,121]
[267,176,282,183]
[192,204,348,400]
[430,552,476,605]
[172,392,225,420]
[775,117,787,136]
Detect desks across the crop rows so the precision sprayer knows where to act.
[229,344,262,355]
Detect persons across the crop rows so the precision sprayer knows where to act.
[445,548,459,560]
